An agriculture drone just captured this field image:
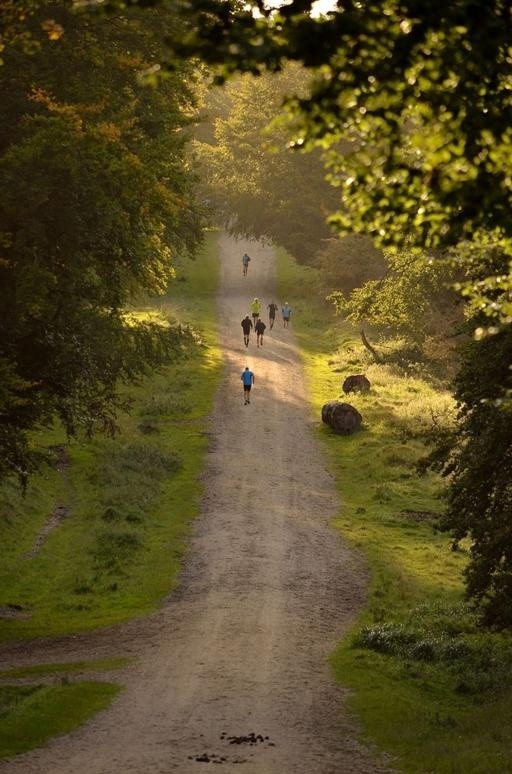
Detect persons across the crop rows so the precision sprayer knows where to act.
[266,299,278,329]
[254,318,266,348]
[281,301,292,329]
[242,252,250,277]
[240,367,256,406]
[240,315,254,347]
[248,298,264,318]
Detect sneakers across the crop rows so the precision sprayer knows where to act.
[245,343,263,348]
[245,399,250,405]
